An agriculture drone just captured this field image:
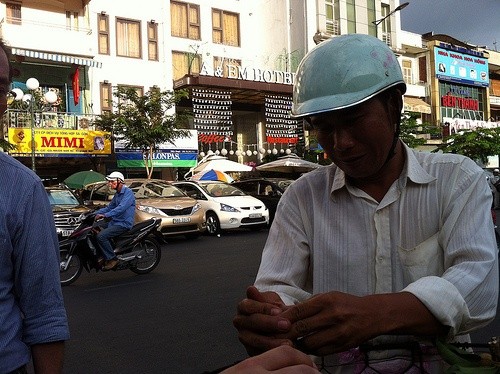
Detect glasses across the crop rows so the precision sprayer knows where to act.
[0,88,16,105]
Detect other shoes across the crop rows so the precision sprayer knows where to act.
[101,260,118,271]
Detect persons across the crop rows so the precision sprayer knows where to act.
[236,34,500,374]
[0,40,72,373]
[221,345,322,374]
[90,171,136,271]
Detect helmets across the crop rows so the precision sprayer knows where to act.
[105,172,124,181]
[291,34,406,118]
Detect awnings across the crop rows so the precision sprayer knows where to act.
[404,97,431,114]
[12,48,102,69]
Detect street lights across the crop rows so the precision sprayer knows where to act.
[372,2,409,39]
[11,78,58,174]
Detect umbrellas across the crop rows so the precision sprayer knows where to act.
[255,153,321,174]
[191,169,236,184]
[194,155,254,173]
[63,168,107,190]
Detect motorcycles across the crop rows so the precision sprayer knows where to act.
[59,205,168,287]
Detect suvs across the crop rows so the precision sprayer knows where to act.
[44,179,295,254]
